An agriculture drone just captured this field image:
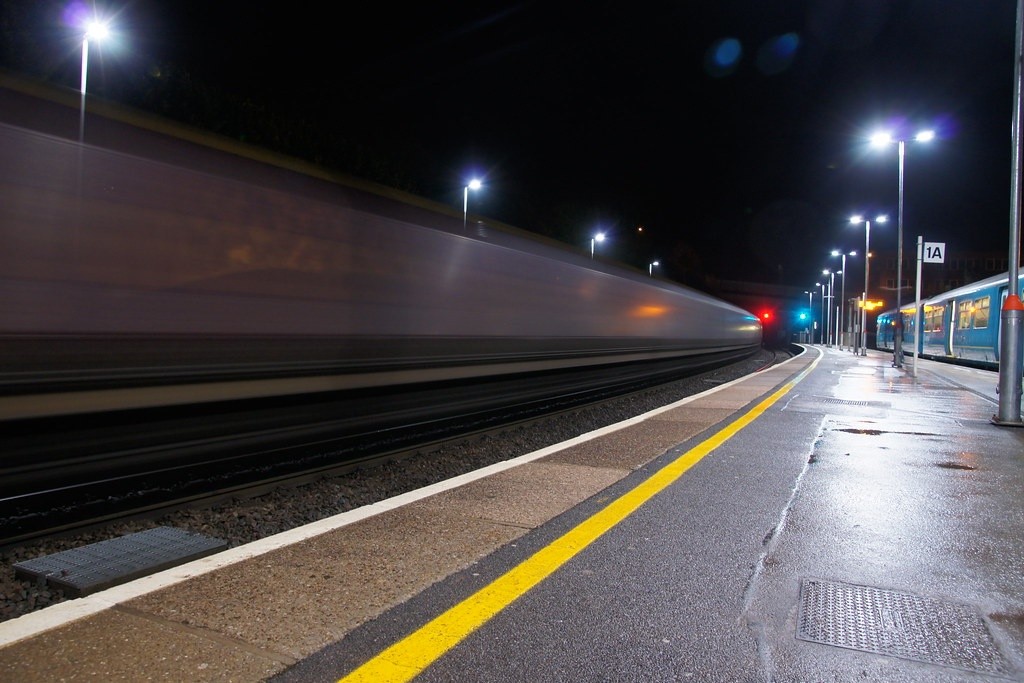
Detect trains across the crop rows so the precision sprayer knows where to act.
[876,265,1024,374]
[1,66,763,494]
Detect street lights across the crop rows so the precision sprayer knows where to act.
[590,230,607,261]
[816,282,830,346]
[805,291,817,346]
[78,19,112,145]
[831,250,856,352]
[849,215,888,356]
[462,177,481,232]
[822,269,843,348]
[869,130,937,367]
